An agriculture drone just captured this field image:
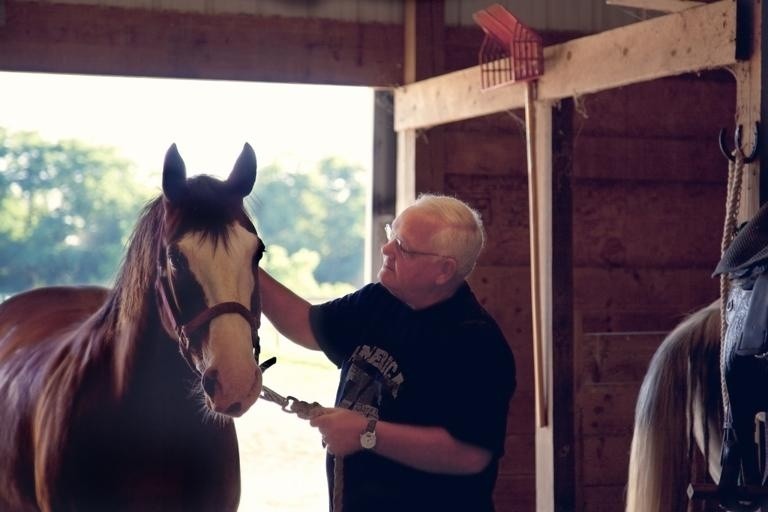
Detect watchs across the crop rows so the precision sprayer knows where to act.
[359,415,378,450]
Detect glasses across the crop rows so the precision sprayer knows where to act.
[384,222,441,258]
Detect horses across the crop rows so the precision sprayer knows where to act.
[621,297,768,511]
[0,141,269,511]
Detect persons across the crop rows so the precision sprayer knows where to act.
[253,190,518,512]
